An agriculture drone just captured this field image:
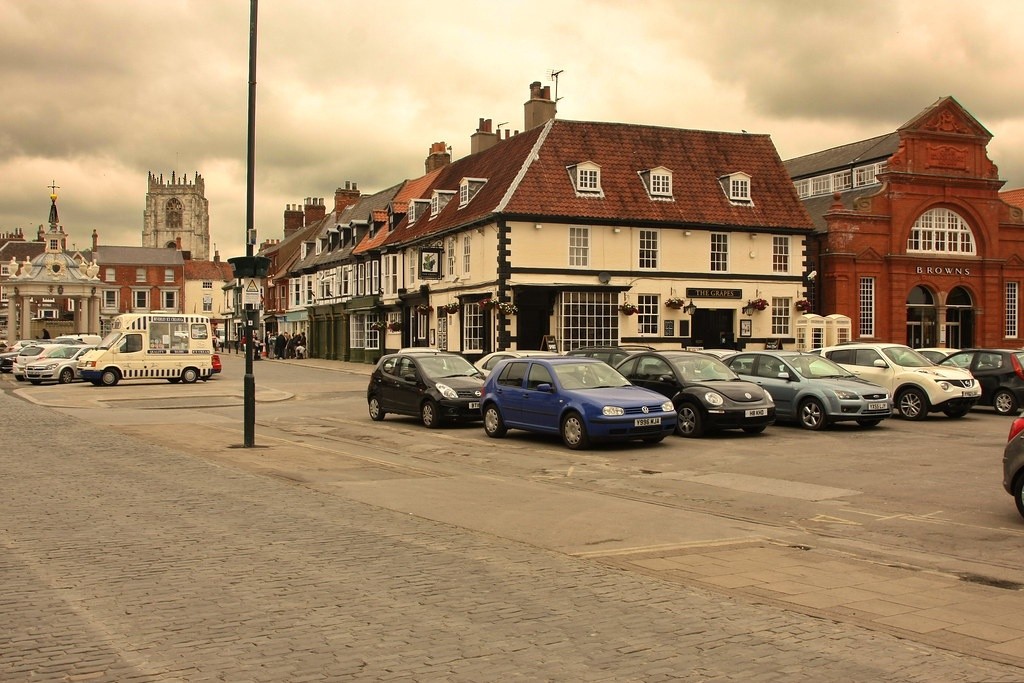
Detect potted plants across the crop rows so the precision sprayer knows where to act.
[665,297,684,309]
[620,303,639,315]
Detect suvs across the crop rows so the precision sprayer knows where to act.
[1002,411,1024,519]
[474,345,658,379]
[820,341,992,420]
[936,348,1024,416]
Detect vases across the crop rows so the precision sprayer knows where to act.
[500,310,511,315]
[392,327,400,331]
[483,305,492,310]
[378,327,383,331]
[447,309,457,314]
[420,310,428,314]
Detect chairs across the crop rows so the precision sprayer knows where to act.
[979,355,992,368]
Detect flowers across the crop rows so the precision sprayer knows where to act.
[371,320,387,329]
[752,299,769,310]
[479,297,498,307]
[796,300,812,311]
[417,304,434,312]
[443,303,460,309]
[389,322,402,328]
[498,303,519,313]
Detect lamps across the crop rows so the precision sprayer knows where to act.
[742,300,755,316]
[683,299,696,315]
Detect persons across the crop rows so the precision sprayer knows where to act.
[214,331,308,360]
[34,328,50,340]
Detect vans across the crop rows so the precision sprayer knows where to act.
[76,310,214,386]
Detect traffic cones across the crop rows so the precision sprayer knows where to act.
[262,345,267,356]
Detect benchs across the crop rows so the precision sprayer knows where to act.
[732,361,753,372]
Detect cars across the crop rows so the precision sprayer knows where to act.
[720,347,895,432]
[367,347,488,428]
[614,349,776,437]
[0,332,102,385]
[479,355,678,450]
